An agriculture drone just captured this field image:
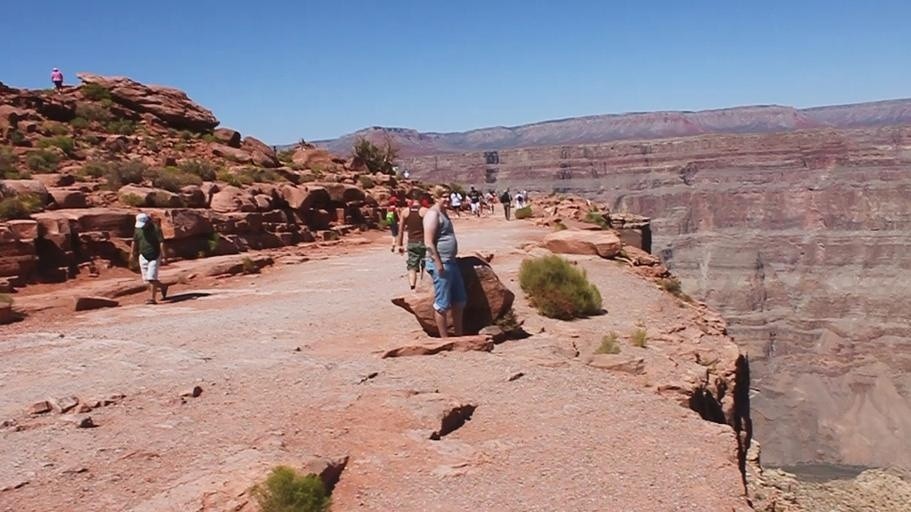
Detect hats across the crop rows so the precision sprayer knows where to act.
[135,213,149,229]
[389,196,401,203]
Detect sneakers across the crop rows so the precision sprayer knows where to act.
[144,284,168,304]
[391,245,396,253]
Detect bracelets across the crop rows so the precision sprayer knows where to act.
[160,255,167,260]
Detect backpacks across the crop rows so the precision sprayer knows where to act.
[387,207,397,226]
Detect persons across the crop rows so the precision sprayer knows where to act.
[397,189,432,289]
[419,183,468,339]
[500,192,513,220]
[515,191,525,210]
[384,196,402,254]
[273,146,277,156]
[51,68,64,90]
[126,212,169,305]
[450,185,498,218]
